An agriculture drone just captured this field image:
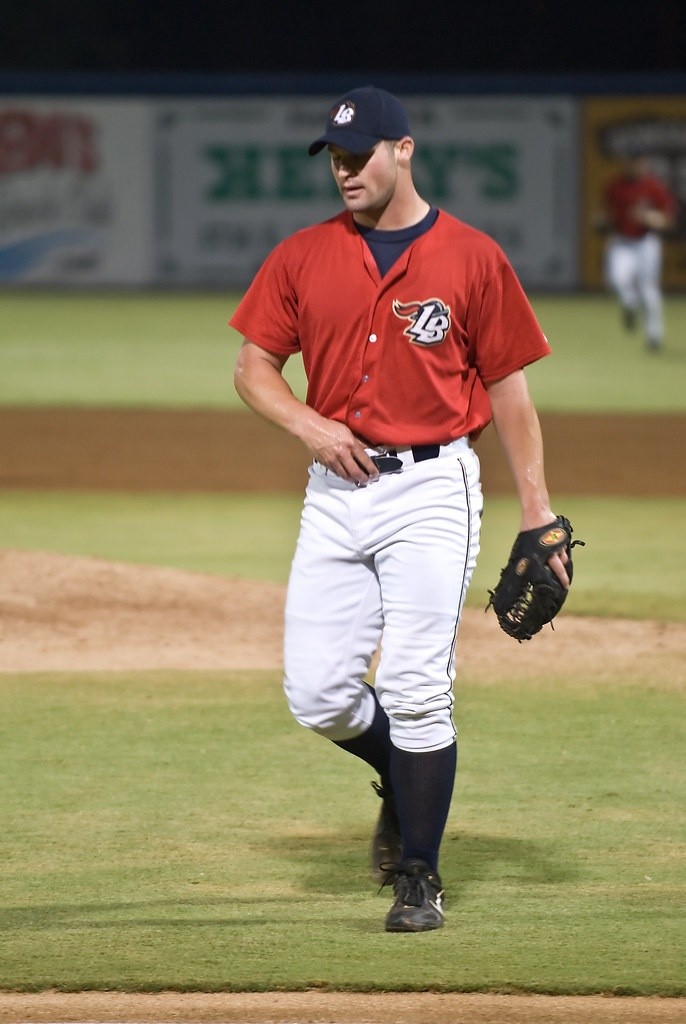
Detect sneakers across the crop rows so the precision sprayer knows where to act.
[368,779,401,884]
[375,858,445,931]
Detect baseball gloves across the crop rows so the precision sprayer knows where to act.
[483,514,587,645]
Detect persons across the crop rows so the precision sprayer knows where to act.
[593,152,675,356]
[226,86,567,933]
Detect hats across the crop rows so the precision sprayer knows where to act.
[308,87,410,157]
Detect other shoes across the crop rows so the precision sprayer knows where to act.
[619,304,635,330]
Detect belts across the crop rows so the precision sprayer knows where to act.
[357,444,440,475]
